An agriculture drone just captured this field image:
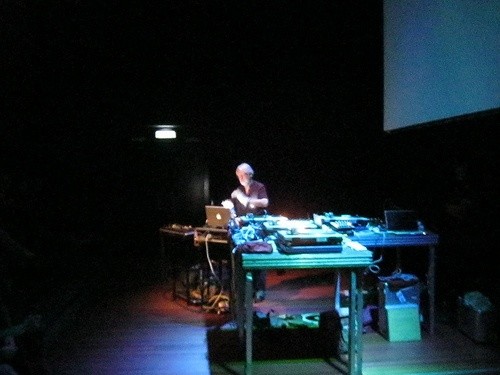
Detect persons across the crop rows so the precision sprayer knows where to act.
[231,163,269,303]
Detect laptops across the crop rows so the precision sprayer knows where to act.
[205,206,231,228]
[385,210,426,235]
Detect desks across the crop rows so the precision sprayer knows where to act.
[161,230,211,305]
[321,214,439,338]
[228,221,372,375]
[198,238,228,311]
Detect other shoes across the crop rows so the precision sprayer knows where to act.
[255,290,264,303]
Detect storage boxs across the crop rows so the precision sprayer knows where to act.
[456,305,499,344]
[378,281,422,343]
